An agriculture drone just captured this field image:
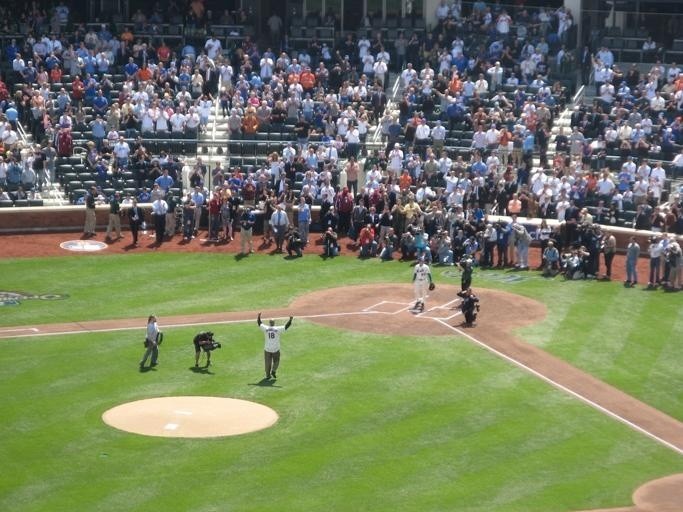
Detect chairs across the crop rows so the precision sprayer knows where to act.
[1,0,683,233]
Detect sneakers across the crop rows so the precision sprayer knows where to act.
[264,371,277,383]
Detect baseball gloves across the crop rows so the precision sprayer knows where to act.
[429,283,435,290]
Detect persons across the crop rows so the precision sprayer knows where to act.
[137,314,163,370]
[457,258,473,296]
[410,256,434,310]
[191,330,223,369]
[255,312,293,382]
[456,287,481,327]
